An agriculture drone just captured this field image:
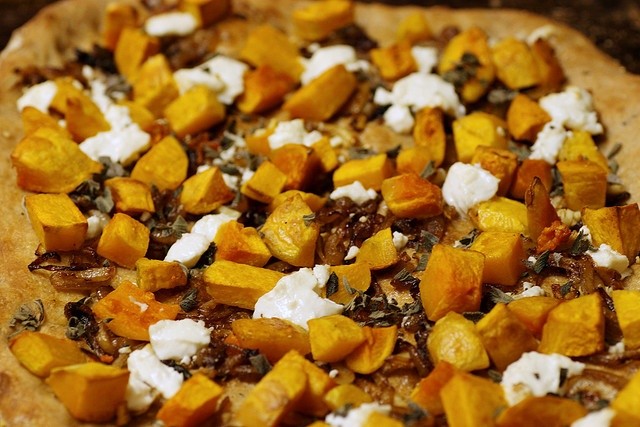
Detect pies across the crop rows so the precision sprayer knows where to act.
[1,1,640,427]
